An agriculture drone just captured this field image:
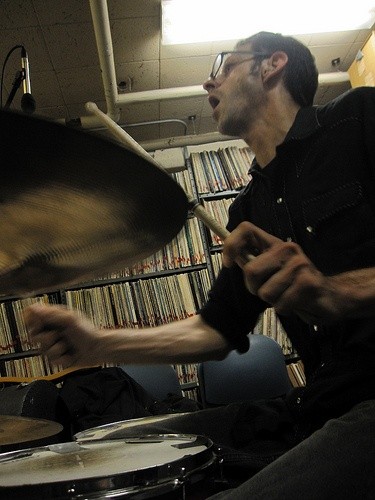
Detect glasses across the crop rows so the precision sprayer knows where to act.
[207,49,275,81]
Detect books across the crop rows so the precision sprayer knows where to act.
[170,363,199,403]
[0,294,63,388]
[203,198,236,280]
[172,146,255,199]
[66,269,211,367]
[93,217,207,281]
[250,307,306,388]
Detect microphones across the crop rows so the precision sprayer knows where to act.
[20,47,37,115]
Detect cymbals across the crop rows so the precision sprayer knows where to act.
[0,414,65,446]
[0,104,189,300]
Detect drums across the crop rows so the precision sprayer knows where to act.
[0,432,224,500]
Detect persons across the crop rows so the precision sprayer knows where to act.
[26,32,375,500]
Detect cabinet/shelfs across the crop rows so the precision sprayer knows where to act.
[1,147,300,391]
